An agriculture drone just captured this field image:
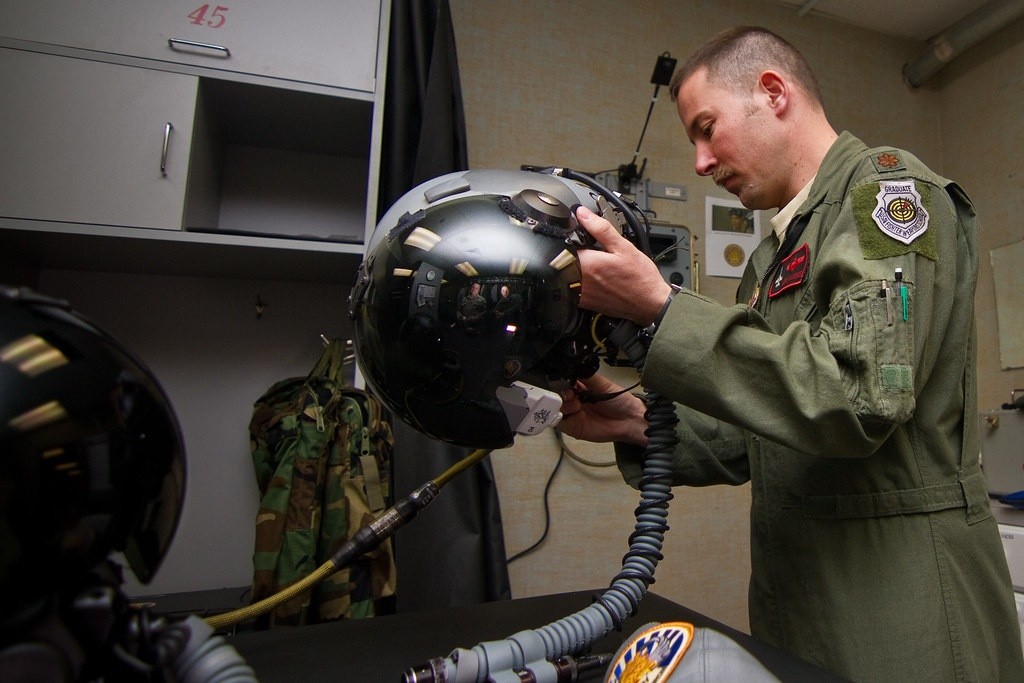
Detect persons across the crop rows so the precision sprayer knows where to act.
[543,23,1023,683]
[456,283,486,320]
[495,284,525,319]
[724,207,753,233]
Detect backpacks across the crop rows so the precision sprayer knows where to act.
[247,336,399,628]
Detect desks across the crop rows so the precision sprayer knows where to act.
[2,586,853,680]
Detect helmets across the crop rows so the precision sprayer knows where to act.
[1,285,186,683]
[346,164,654,452]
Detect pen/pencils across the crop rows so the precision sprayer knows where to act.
[881,278,888,298]
[894,267,903,296]
[900,285,909,320]
[885,287,893,326]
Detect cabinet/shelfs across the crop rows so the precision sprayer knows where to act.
[0,2,393,258]
